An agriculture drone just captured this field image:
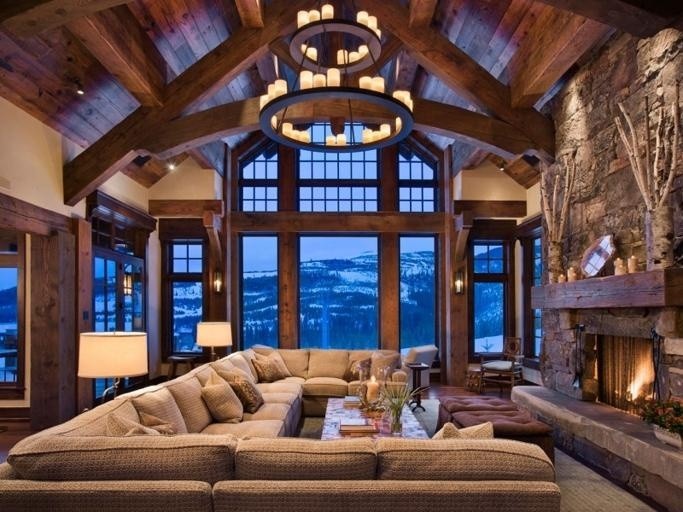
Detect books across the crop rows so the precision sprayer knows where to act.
[343,396,362,409]
[339,417,379,437]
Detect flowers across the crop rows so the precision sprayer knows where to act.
[639,395,683,432]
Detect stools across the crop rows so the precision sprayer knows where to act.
[434,395,556,470]
[166,354,201,380]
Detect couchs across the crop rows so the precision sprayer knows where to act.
[0,344,560,512]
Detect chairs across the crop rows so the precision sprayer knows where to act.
[481,336,523,398]
[396,346,439,391]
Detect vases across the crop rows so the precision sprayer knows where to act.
[654,423,683,448]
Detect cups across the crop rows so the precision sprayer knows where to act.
[628,258,638,273]
[567,269,576,282]
[614,260,624,276]
[557,275,566,283]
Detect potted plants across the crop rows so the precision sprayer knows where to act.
[377,368,432,437]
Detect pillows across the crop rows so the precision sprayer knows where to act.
[431,421,494,441]
[107,348,395,438]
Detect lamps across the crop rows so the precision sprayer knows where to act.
[453,270,466,296]
[258,1,414,154]
[78,330,148,404]
[212,252,222,294]
[196,322,233,362]
[123,271,133,296]
